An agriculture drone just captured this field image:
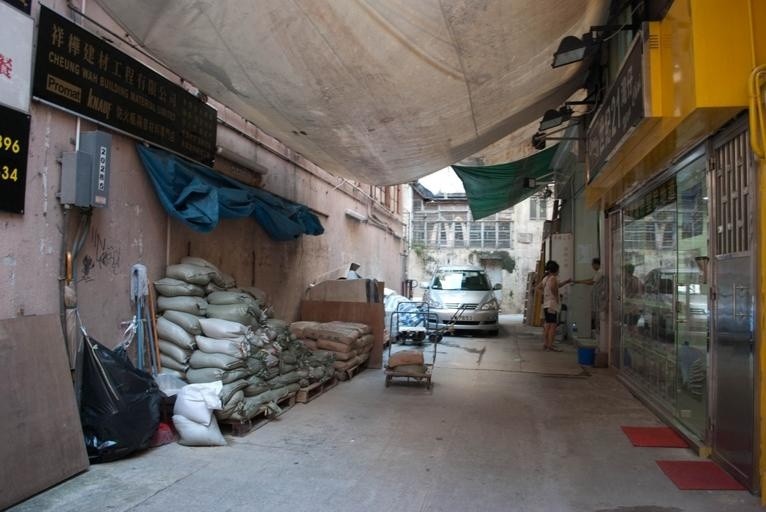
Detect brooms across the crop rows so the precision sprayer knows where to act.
[141,301,174,447]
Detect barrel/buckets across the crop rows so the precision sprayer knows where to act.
[577,345,594,365]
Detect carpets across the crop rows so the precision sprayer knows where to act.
[658,460,747,490]
[621,425,690,448]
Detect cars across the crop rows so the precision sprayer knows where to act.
[642,267,709,345]
[421,265,502,336]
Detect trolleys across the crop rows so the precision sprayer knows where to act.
[429,304,468,343]
[383,312,438,390]
[397,302,429,346]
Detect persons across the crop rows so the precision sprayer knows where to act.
[624,264,645,325]
[571,258,602,338]
[534,260,572,353]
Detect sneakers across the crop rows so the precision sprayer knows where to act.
[544,345,562,352]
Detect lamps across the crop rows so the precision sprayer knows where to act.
[552,23,633,69]
[523,177,555,188]
[539,98,589,129]
